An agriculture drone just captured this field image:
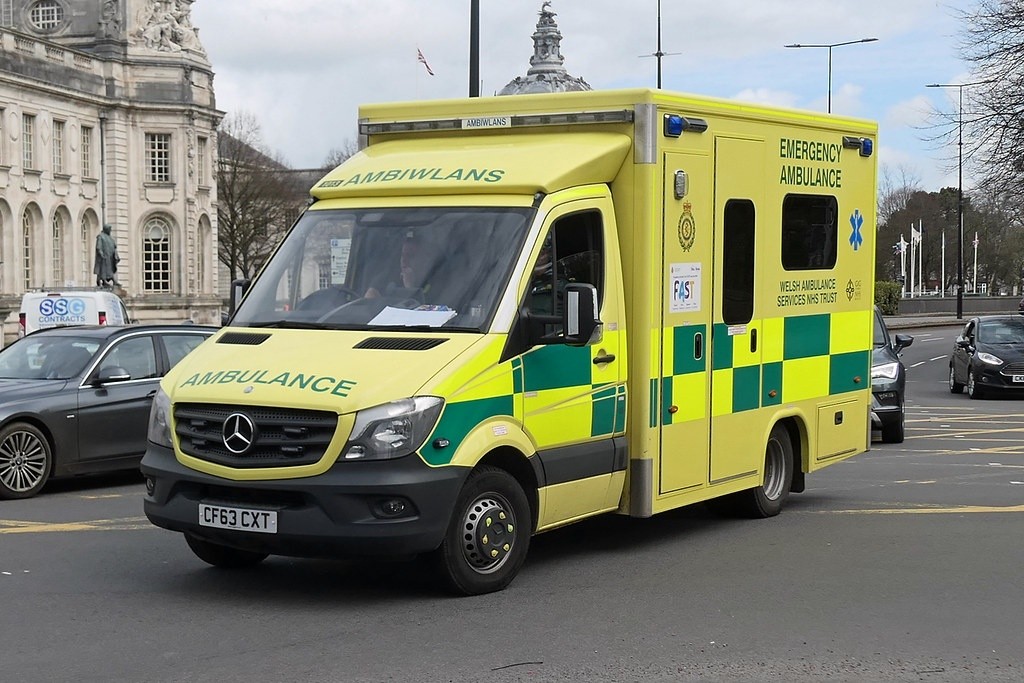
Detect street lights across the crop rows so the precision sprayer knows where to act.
[784,38,879,113]
[926,79,998,319]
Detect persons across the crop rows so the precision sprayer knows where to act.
[94,225,121,286]
[146,3,196,48]
[364,236,444,305]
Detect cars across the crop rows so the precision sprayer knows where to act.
[0,325,223,498]
[1018,298,1024,316]
[949,315,1024,400]
[871,305,914,443]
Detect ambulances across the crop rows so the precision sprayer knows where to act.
[139,87,878,594]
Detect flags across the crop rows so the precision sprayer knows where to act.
[418,48,435,77]
[892,230,920,256]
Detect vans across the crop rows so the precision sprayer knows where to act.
[19,292,140,372]
